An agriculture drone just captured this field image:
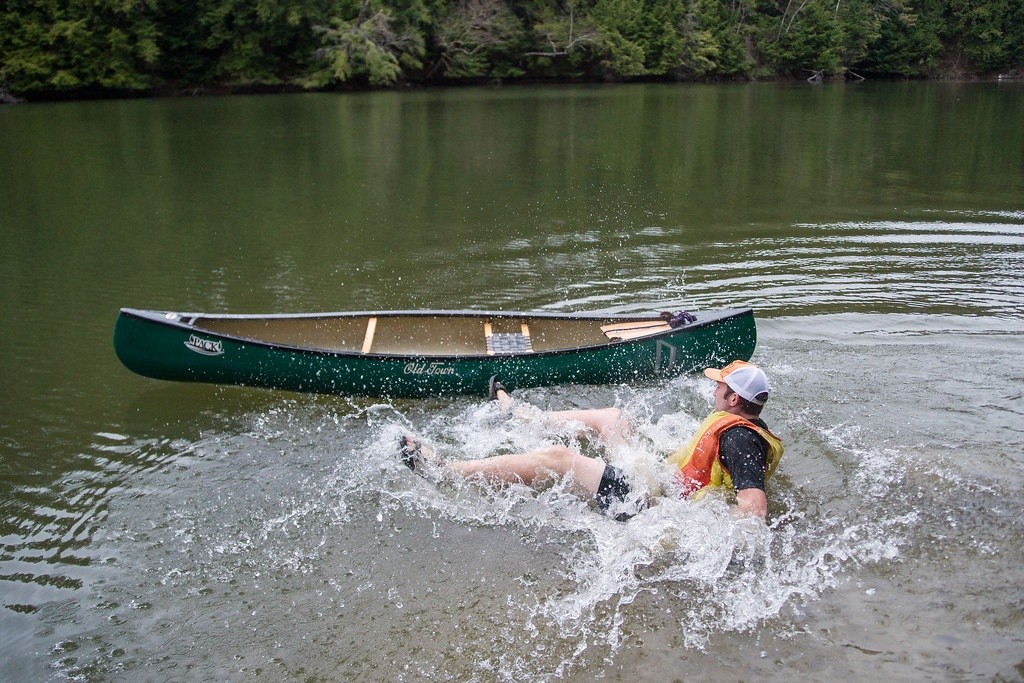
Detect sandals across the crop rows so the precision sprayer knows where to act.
[400,436,457,500]
[487,375,518,429]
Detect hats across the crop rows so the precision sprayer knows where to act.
[704,360,769,405]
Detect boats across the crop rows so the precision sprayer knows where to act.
[111,305,771,402]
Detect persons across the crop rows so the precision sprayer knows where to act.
[402,360,785,519]
